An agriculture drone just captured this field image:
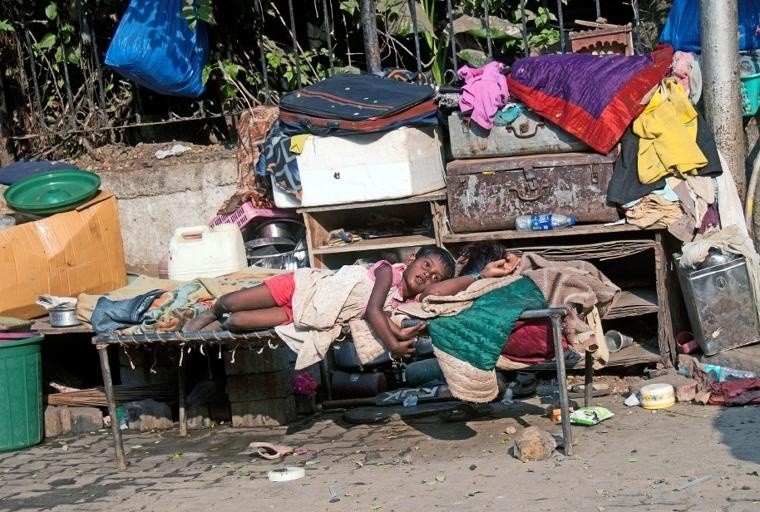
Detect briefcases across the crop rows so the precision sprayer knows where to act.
[446,154,619,234]
[447,106,590,159]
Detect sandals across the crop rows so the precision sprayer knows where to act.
[366,212,405,226]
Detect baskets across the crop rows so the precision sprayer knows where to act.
[208,202,296,228]
[739,65,760,116]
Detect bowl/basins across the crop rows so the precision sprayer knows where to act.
[243,219,307,270]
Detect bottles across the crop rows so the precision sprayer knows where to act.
[516,214,577,231]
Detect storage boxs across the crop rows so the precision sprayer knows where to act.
[264,126,449,211]
[0,188,128,319]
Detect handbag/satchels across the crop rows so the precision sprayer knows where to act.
[276,72,438,136]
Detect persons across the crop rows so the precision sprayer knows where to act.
[418,240,521,303]
[181,244,456,360]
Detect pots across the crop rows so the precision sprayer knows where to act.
[39,295,80,328]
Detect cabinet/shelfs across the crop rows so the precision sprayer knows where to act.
[296,193,675,382]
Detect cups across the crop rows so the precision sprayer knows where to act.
[605,330,633,352]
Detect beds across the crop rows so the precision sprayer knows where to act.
[92,269,571,456]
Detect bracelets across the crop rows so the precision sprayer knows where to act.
[469,271,486,281]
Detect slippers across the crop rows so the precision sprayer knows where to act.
[567,382,610,397]
[440,402,492,421]
[513,373,536,398]
[675,328,699,354]
[248,441,292,460]
